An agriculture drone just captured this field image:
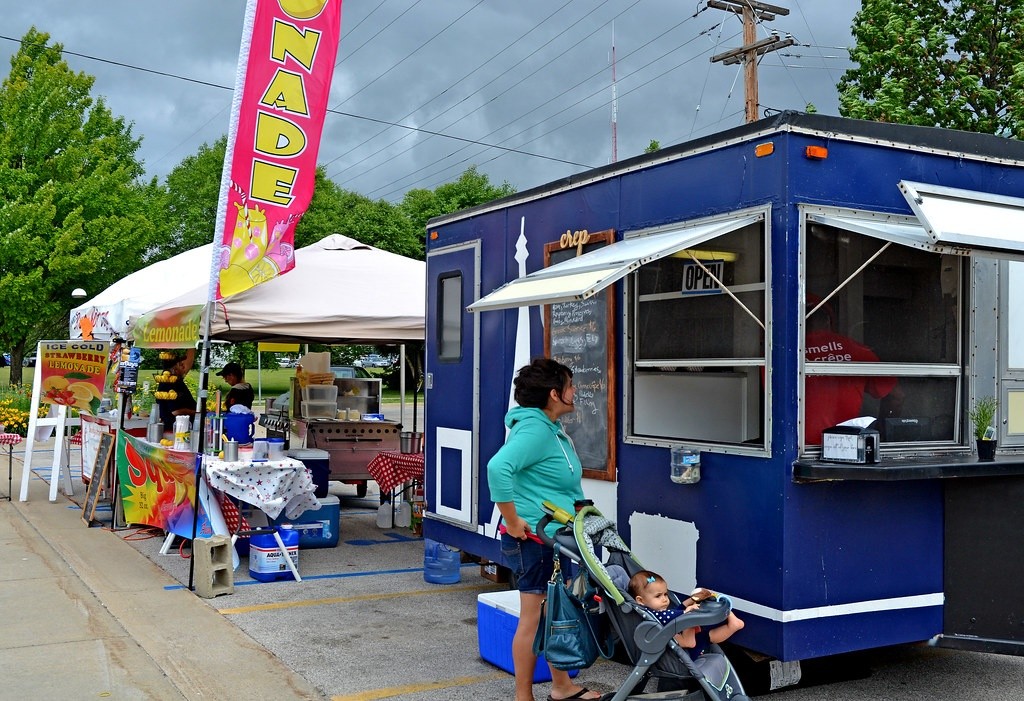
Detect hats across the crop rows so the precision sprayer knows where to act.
[215,362,241,376]
[805,293,834,321]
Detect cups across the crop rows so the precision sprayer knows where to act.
[146,423,165,443]
[223,441,238,462]
[151,404,160,423]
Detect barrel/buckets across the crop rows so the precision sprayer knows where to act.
[249,524,299,583]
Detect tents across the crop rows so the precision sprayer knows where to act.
[69,233,427,591]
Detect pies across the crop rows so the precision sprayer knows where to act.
[310,373,335,382]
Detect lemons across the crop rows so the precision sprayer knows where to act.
[218,451,224,458]
[159,439,173,446]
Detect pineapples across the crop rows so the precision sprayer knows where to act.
[154,352,178,400]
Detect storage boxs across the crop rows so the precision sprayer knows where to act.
[478,589,579,683]
[275,494,341,549]
[235,509,271,559]
[288,448,331,498]
[301,401,337,419]
[301,385,338,403]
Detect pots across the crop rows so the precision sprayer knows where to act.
[400,431,422,454]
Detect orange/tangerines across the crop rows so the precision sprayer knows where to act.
[66,382,101,410]
[42,376,69,391]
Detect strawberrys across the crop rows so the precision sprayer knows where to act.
[43,387,77,405]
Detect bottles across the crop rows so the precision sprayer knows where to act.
[376,500,392,529]
[423,538,461,585]
[396,500,411,528]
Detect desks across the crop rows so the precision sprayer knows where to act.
[366,451,426,529]
[126,435,322,556]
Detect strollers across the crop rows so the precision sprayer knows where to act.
[536,503,755,701]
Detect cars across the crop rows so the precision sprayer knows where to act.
[327,364,372,379]
[275,357,301,368]
[0,351,37,367]
[346,352,398,368]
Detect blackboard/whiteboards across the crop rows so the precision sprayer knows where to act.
[542,228,617,482]
[80,431,115,527]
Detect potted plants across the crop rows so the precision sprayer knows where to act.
[968,395,997,463]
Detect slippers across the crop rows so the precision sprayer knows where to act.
[547,685,602,701]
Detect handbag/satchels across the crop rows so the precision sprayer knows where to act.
[545,541,613,671]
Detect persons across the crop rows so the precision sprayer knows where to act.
[156,347,198,431]
[759,297,906,453]
[215,363,254,433]
[487,357,605,701]
[624,571,744,661]
